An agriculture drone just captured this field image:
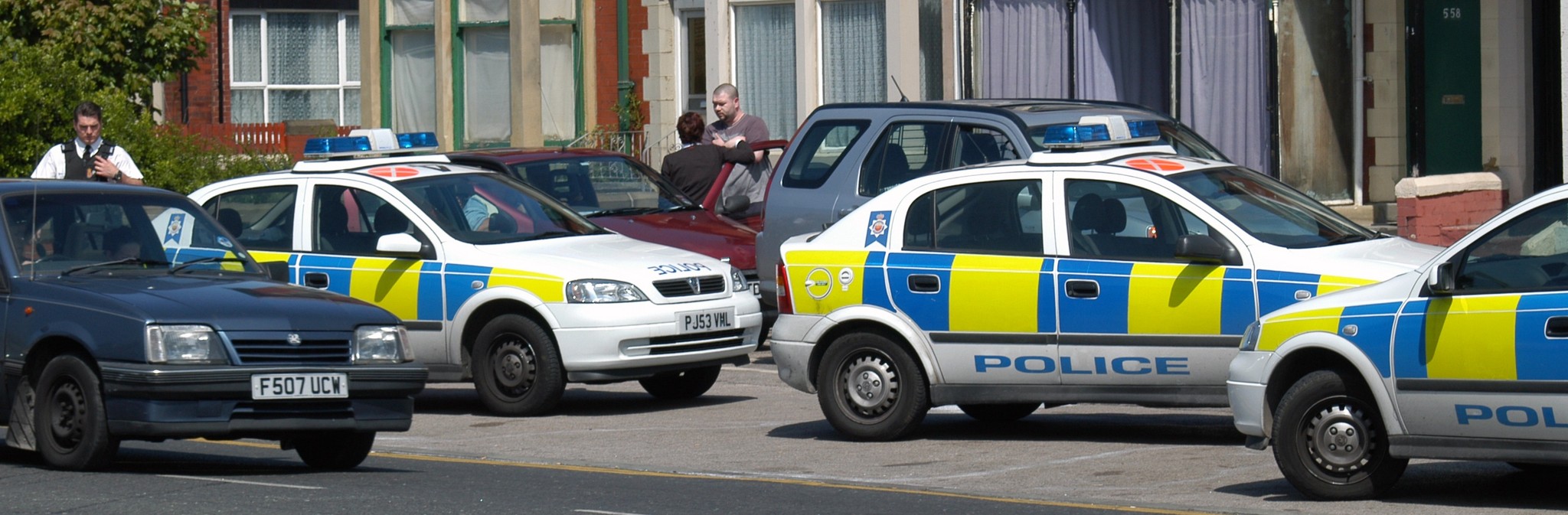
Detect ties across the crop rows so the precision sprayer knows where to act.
[82,145,91,159]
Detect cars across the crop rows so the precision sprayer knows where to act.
[438,138,791,313]
[150,127,764,418]
[0,176,430,473]
[767,114,1486,444]
[1225,180,1568,503]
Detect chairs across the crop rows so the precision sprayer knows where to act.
[908,201,943,248]
[212,208,244,245]
[1069,193,1127,257]
[965,206,1024,253]
[374,203,409,237]
[872,142,910,195]
[320,201,364,254]
[961,131,999,165]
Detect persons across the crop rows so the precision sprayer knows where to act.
[700,83,778,218]
[108,226,143,265]
[659,112,755,214]
[31,100,145,264]
[462,162,526,234]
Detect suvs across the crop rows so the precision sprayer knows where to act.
[753,97,1240,332]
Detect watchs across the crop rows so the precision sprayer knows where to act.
[113,169,122,182]
